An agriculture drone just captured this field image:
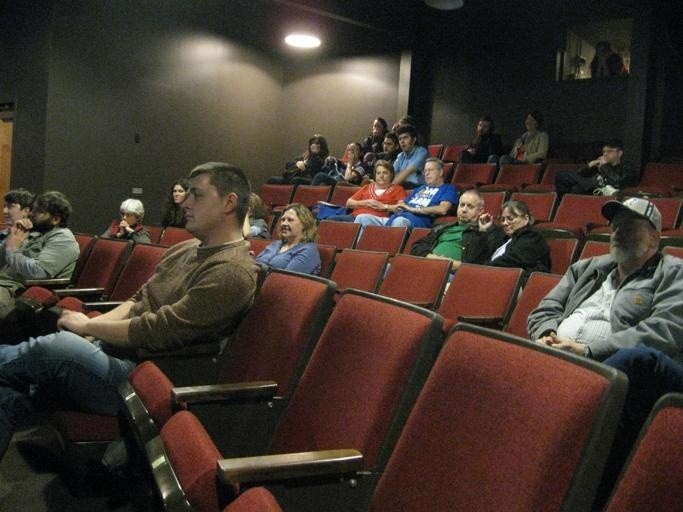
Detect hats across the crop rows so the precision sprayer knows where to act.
[601,197,662,233]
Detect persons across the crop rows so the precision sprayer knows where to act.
[242,114,459,244]
[1,190,80,320]
[526,197,683,450]
[589,40,629,75]
[1,162,257,454]
[458,110,636,200]
[110,179,190,245]
[467,202,551,281]
[252,204,321,282]
[409,190,498,268]
[1,189,33,268]
